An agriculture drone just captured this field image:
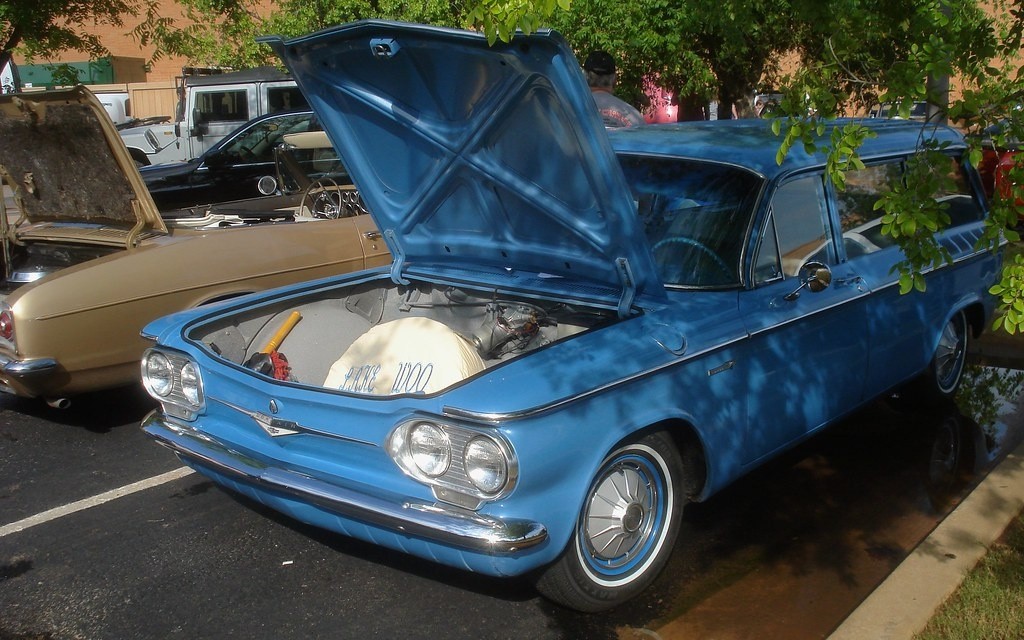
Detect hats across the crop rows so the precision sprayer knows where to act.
[579,50,615,76]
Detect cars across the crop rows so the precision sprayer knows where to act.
[140,21,1007,620]
[0,70,649,411]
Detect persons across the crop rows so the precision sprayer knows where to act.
[584,50,645,129]
